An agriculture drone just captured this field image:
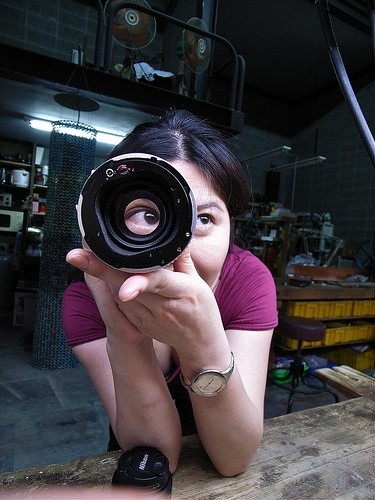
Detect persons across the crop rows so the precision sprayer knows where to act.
[61,107,279,479]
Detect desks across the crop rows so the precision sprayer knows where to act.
[0,365,375,500]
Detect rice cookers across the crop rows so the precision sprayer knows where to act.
[10,169,29,188]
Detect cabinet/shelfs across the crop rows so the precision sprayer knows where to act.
[0,133,51,322]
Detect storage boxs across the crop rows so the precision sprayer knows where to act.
[277,300,375,371]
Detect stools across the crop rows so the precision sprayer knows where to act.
[278,315,339,413]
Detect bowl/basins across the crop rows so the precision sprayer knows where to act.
[288,273,312,287]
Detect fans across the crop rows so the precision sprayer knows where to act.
[177,17,211,99]
[104,0,156,81]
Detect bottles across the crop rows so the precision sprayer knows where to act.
[31,193,39,213]
[34,168,43,184]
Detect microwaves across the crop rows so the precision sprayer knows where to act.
[0,193,12,207]
[0,210,23,232]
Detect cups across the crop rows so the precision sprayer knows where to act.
[111,446,172,500]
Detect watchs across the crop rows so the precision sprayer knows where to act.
[180,351,236,399]
